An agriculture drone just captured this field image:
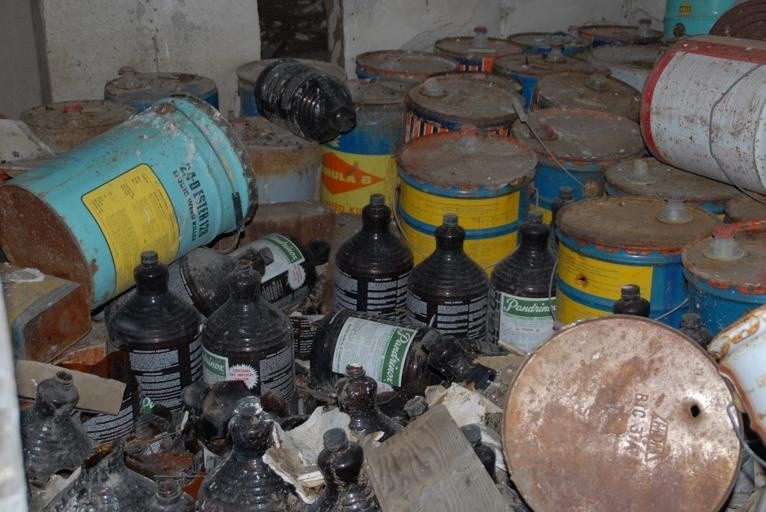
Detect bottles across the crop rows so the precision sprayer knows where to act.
[20,193,660,512]
[254,56,358,143]
